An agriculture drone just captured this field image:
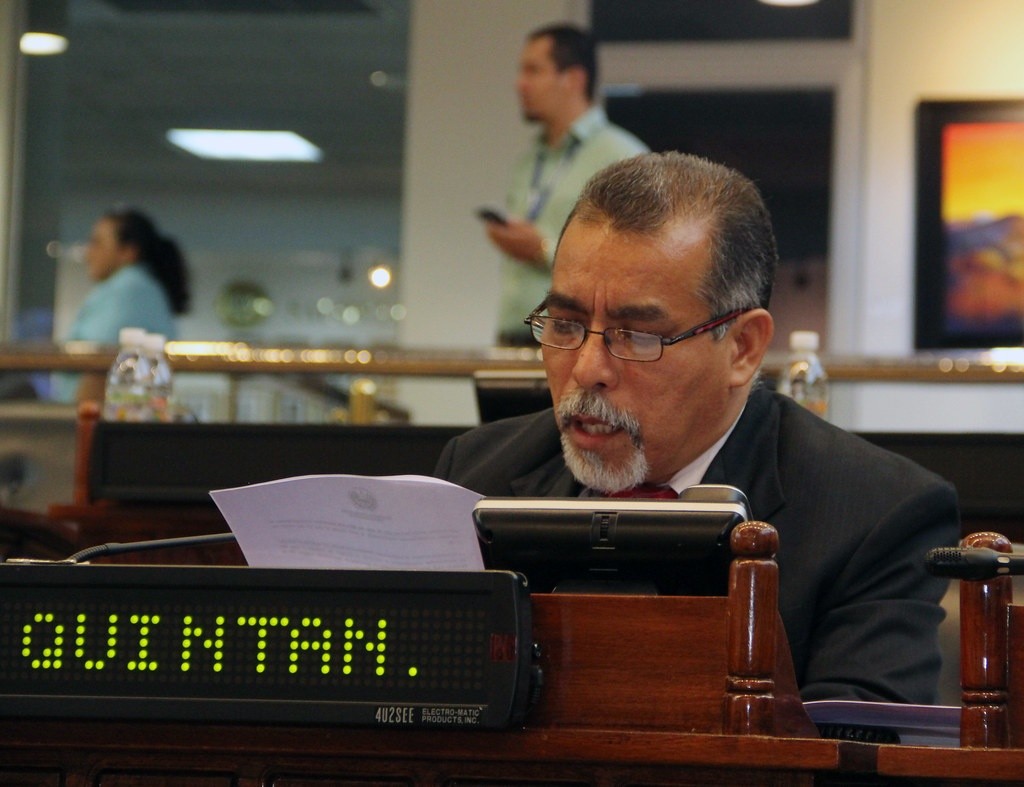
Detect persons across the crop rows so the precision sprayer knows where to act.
[49,206,186,402]
[474,22,648,421]
[431,153,962,741]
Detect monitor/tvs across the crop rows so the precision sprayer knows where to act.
[473,484,752,597]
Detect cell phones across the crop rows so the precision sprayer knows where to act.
[479,208,505,226]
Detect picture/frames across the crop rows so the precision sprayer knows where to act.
[915,98,1024,350]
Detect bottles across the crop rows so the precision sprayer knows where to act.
[104,329,147,422]
[138,334,174,421]
[780,331,830,421]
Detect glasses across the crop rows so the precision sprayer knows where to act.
[524,296,761,363]
